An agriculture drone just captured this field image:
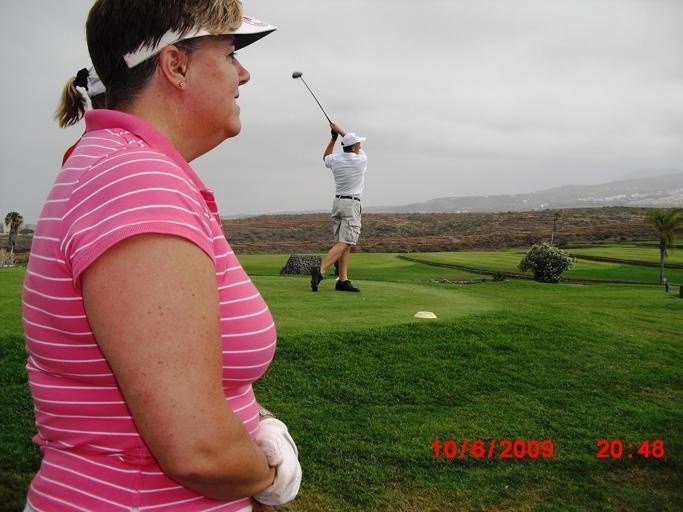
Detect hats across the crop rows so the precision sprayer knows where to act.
[86,66,106,99]
[340,132,365,148]
[98,10,278,87]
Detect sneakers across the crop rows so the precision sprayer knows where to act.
[311,266,324,292]
[335,279,360,293]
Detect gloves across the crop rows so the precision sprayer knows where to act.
[253,418,302,506]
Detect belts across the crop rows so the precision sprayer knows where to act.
[336,195,361,201]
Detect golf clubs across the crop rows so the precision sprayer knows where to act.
[292,72,334,125]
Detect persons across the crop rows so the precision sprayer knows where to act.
[22,0,301,511]
[309,122,368,292]
